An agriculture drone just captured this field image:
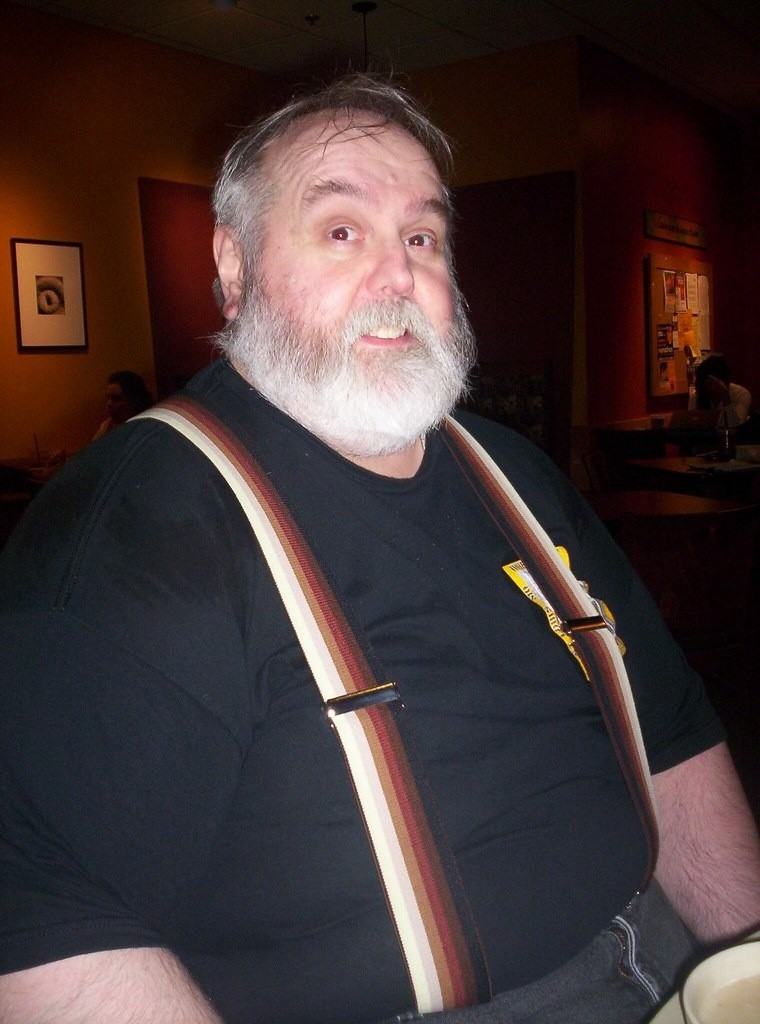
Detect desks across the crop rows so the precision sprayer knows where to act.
[627,456,760,475]
[586,490,760,520]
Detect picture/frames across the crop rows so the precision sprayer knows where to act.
[10,237,89,350]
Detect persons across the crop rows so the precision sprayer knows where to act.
[1,72,760,1023]
[93,369,156,436]
[688,354,752,432]
